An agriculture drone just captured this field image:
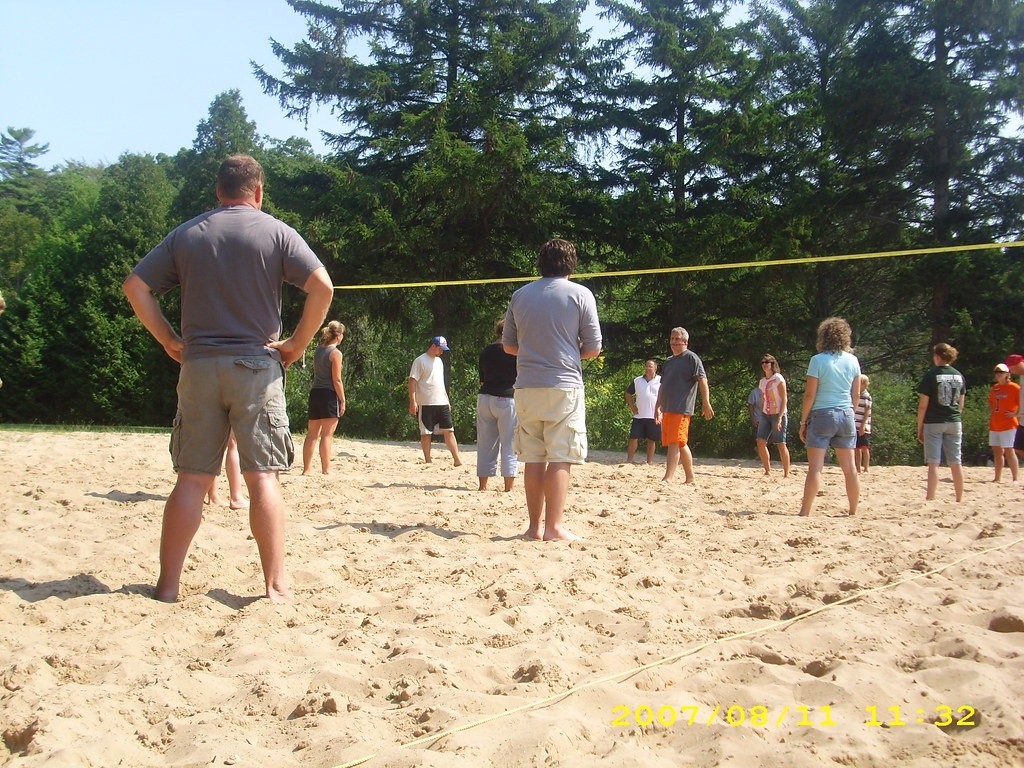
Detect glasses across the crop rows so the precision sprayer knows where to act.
[761,361,772,364]
[994,371,1006,373]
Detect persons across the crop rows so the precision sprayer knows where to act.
[477,319,519,492]
[1005,354,1024,456]
[854,374,872,473]
[799,317,861,516]
[123,156,333,601]
[749,388,760,460]
[917,343,966,502]
[988,363,1020,481]
[757,353,790,477]
[409,336,463,466]
[303,320,345,475]
[654,327,714,483]
[206,430,250,510]
[502,239,602,541]
[625,360,661,463]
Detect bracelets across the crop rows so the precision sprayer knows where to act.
[800,421,807,426]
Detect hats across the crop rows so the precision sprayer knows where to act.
[430,336,450,351]
[1007,354,1024,366]
